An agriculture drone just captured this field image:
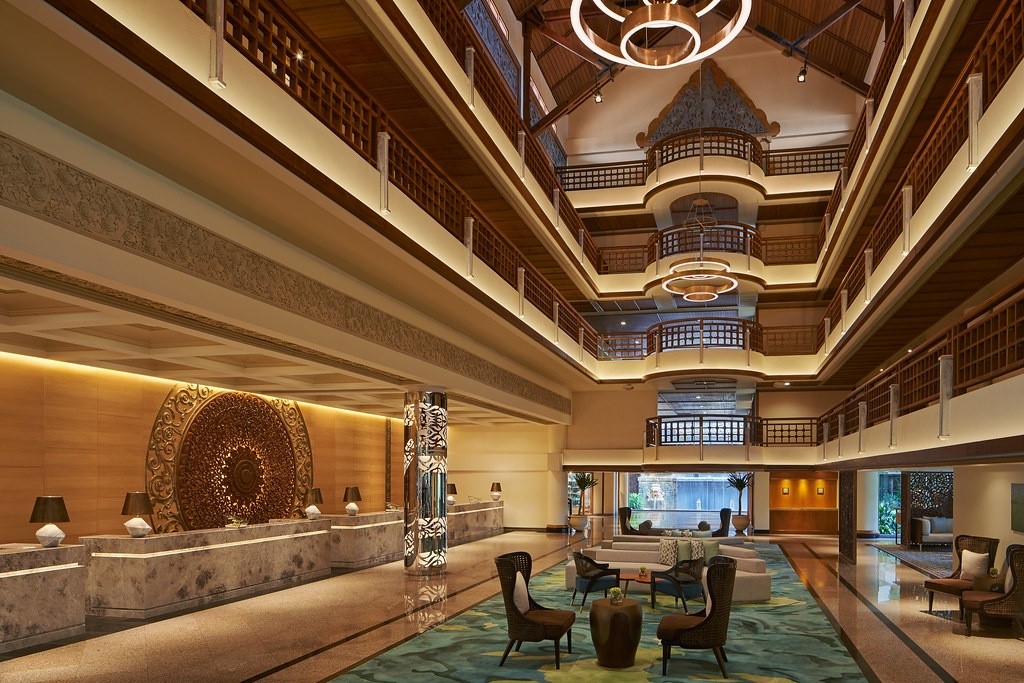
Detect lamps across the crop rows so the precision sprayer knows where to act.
[29,496,70,547]
[491,483,502,501]
[121,491,155,537]
[662,61,738,303]
[305,488,325,519]
[343,486,362,516]
[569,0,753,69]
[797,48,810,82]
[594,72,602,103]
[446,483,458,505]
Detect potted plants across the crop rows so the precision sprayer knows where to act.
[609,587,622,605]
[725,472,757,536]
[639,567,648,578]
[569,473,599,530]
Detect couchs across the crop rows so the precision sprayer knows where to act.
[912,516,953,551]
[566,542,772,603]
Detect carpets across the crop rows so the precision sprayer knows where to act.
[871,543,953,579]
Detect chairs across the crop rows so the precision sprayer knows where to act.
[618,507,638,535]
[713,508,731,537]
[570,552,620,613]
[651,558,709,613]
[959,544,1024,637]
[657,555,737,679]
[494,552,576,670]
[924,535,1000,620]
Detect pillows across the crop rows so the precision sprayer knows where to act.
[961,549,990,581]
[659,539,719,566]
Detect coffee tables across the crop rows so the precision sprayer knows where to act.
[589,598,642,668]
[608,572,672,602]
